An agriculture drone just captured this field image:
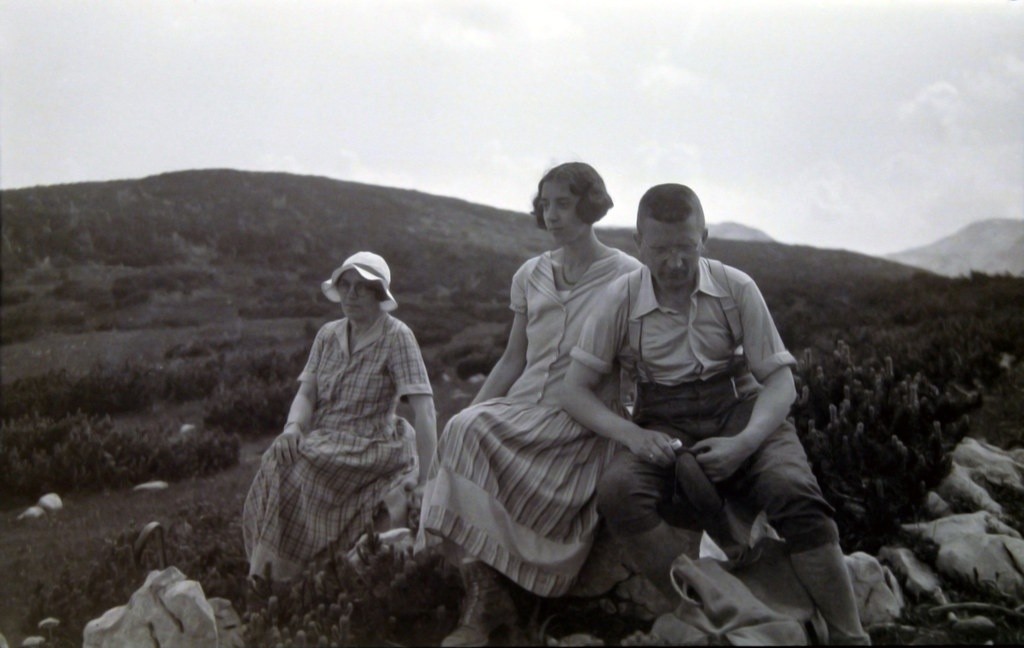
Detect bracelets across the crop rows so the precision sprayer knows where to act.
[284,421,304,431]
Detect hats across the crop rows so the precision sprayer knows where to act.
[321,251,398,311]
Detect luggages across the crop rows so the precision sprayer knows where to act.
[133,522,242,648]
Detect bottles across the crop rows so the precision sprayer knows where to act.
[670,438,690,456]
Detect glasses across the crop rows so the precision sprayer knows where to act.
[338,279,377,298]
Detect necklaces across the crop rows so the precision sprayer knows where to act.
[562,249,590,285]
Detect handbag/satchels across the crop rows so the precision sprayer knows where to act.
[670,551,807,646]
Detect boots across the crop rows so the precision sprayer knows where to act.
[623,520,687,612]
[790,542,871,646]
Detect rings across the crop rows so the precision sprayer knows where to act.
[648,454,654,461]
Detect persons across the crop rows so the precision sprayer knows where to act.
[241,250,437,599]
[412,163,646,648]
[559,185,874,648]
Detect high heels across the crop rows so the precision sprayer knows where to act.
[442,561,520,648]
